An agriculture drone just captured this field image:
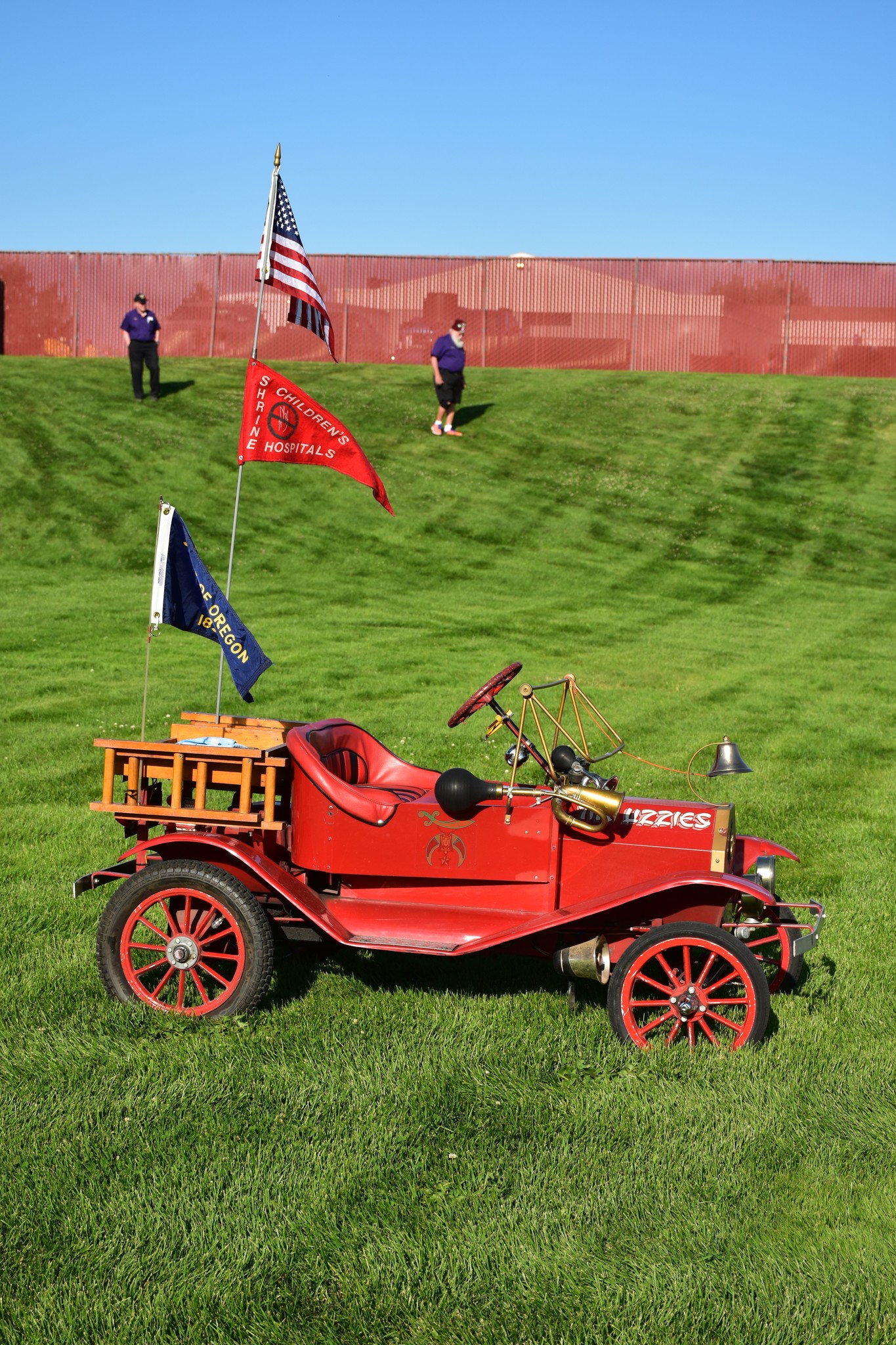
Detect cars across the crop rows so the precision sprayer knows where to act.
[74,661,826,1067]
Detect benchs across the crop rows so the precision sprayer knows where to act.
[285,718,442,827]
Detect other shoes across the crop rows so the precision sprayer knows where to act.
[442,426,463,437]
[430,424,442,435]
[151,390,159,401]
[135,392,147,402]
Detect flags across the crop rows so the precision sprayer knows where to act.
[149,501,272,704]
[236,356,395,518]
[254,168,341,365]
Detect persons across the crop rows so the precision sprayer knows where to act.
[121,293,161,401]
[430,319,467,437]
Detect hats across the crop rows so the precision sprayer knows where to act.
[134,293,148,304]
[452,319,466,331]
